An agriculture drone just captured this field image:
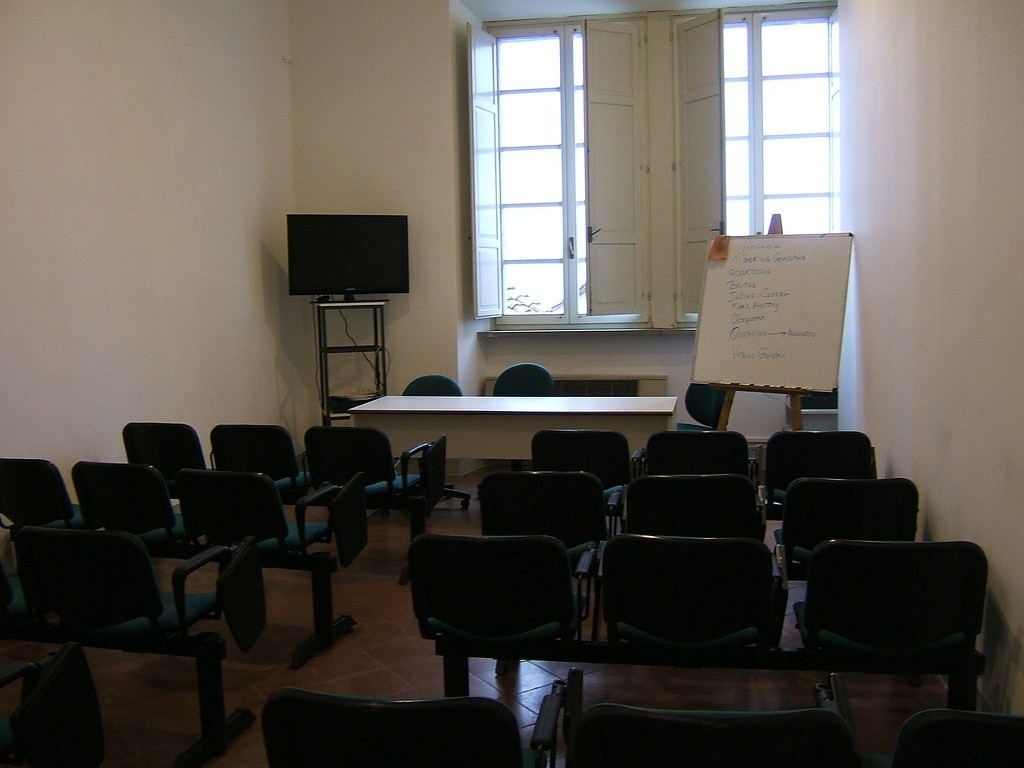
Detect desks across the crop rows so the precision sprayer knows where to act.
[346,395,679,463]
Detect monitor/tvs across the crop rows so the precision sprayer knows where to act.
[286,214,409,303]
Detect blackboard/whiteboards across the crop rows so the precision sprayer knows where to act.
[689,232,853,394]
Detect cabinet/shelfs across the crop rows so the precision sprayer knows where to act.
[310,300,391,427]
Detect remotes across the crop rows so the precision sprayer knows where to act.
[316,296,330,301]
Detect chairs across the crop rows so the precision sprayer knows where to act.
[0,362,1024,768]
